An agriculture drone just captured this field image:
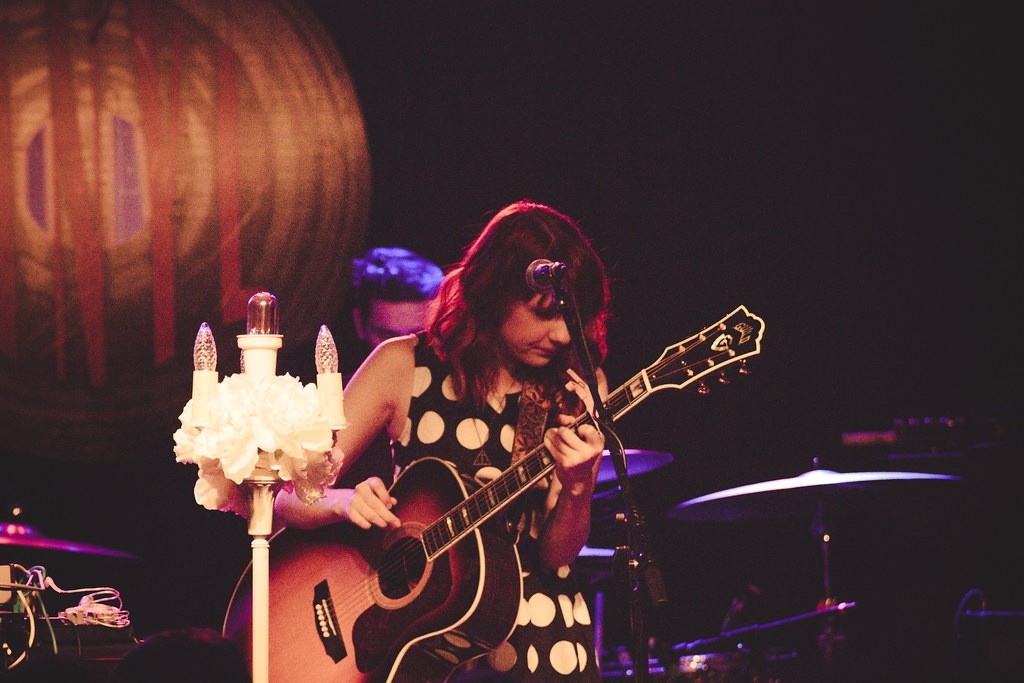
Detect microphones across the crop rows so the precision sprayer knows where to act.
[526,259,567,293]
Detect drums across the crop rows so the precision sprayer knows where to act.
[670,649,768,683]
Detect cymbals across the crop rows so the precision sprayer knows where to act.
[0,524,137,562]
[665,465,964,525]
[575,547,642,560]
[596,448,672,485]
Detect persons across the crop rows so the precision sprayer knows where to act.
[268,202,621,683]
[343,240,454,368]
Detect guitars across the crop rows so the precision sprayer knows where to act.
[222,305,766,683]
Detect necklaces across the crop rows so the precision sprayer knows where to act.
[460,360,524,466]
